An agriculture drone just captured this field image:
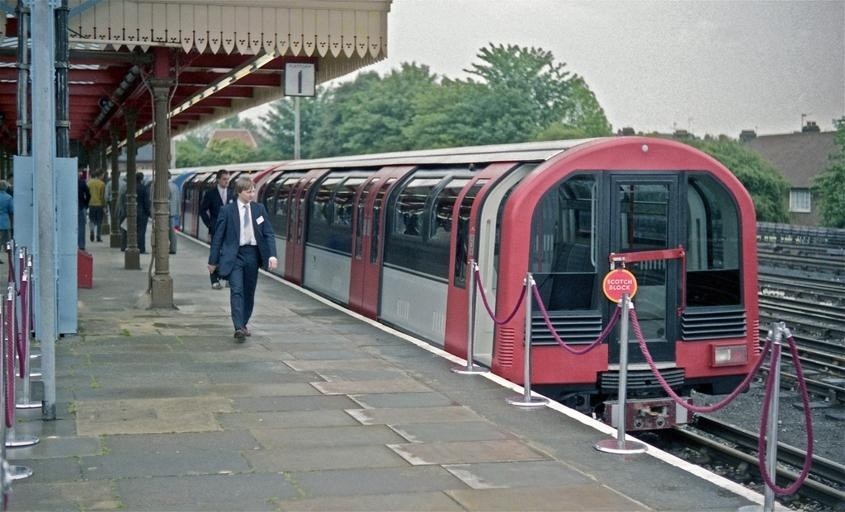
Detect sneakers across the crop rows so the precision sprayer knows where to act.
[212,282,221,289]
[234,326,251,343]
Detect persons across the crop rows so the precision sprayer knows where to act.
[0,179,14,264]
[200,170,234,290]
[168,171,182,254]
[136,171,152,256]
[78,166,127,251]
[206,177,278,340]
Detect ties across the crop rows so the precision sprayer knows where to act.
[243,204,251,245]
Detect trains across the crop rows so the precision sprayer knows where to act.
[118,136,762,449]
